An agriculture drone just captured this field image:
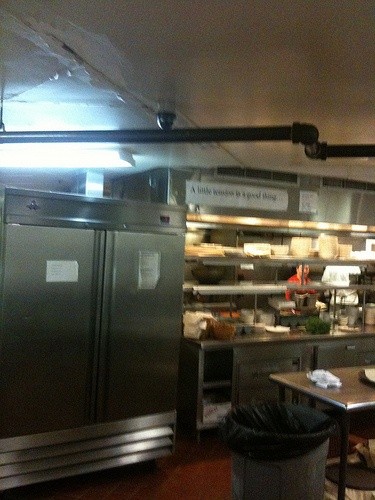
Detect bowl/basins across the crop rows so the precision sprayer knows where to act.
[291,237,312,258]
[211,307,276,339]
[317,234,339,260]
[339,316,348,326]
[338,244,353,260]
[193,267,226,284]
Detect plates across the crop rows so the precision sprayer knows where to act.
[184,232,291,259]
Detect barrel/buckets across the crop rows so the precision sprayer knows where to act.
[364,303,375,325]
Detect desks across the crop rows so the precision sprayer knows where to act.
[269,364,375,500]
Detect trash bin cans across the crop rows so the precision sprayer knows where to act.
[224,402,337,500]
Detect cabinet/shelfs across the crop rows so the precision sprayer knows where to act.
[178,337,375,440]
[183,222,375,294]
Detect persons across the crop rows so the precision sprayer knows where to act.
[285,263,319,312]
[322,265,363,314]
